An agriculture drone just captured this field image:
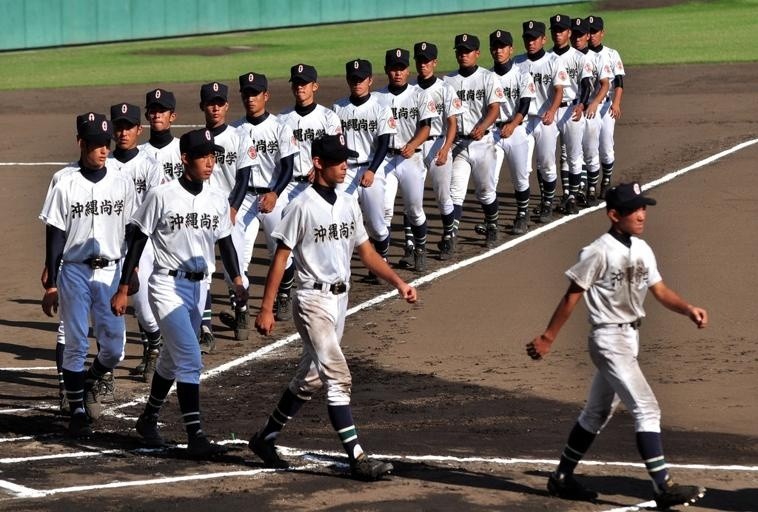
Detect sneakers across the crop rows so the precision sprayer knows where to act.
[219,309,235,329]
[135,414,164,446]
[200,333,216,353]
[566,198,578,214]
[441,237,454,259]
[587,196,598,205]
[84,375,100,419]
[350,458,393,480]
[600,187,610,197]
[654,483,705,511]
[511,211,530,236]
[100,375,114,403]
[234,304,250,340]
[414,250,425,272]
[476,224,487,235]
[485,224,500,248]
[249,433,289,468]
[548,474,596,501]
[437,239,457,250]
[136,356,146,372]
[400,246,415,268]
[276,296,291,321]
[540,201,552,222]
[144,353,159,382]
[557,195,568,211]
[577,190,586,203]
[70,422,93,441]
[57,391,71,415]
[187,443,227,456]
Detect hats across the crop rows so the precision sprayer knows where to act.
[111,103,141,124]
[76,112,106,130]
[179,128,224,158]
[523,20,546,37]
[570,18,589,34]
[606,181,656,215]
[346,60,372,79]
[386,49,409,66]
[585,15,604,31]
[200,82,228,103]
[80,119,113,139]
[146,89,176,112]
[414,43,437,60]
[454,34,479,52]
[548,15,572,32]
[290,63,317,83]
[490,30,512,46]
[239,72,267,92]
[312,133,360,165]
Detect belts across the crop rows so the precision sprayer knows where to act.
[456,131,490,140]
[154,266,210,282]
[429,135,444,140]
[314,281,346,294]
[353,161,369,168]
[292,176,309,182]
[496,116,528,127]
[248,187,270,196]
[595,318,642,329]
[387,148,422,156]
[80,258,123,267]
[560,98,578,107]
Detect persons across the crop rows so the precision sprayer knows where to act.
[107,103,173,383]
[526,180,708,512]
[248,135,417,480]
[474,30,536,234]
[219,73,300,329]
[142,88,184,180]
[331,59,398,285]
[576,16,626,202]
[438,34,506,250]
[110,128,248,457]
[41,112,140,439]
[375,48,439,272]
[199,82,259,354]
[533,14,594,214]
[277,64,343,201]
[512,21,571,223]
[408,41,463,260]
[556,18,614,215]
